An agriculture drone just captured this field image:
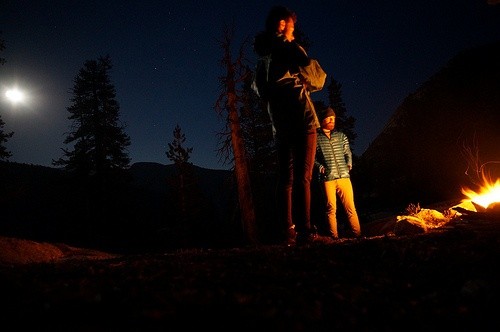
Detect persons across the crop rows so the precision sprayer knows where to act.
[315,109,360,239]
[255,6,334,247]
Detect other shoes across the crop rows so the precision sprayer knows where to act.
[277,225,332,247]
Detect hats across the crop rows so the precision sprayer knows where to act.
[317,107,336,122]
